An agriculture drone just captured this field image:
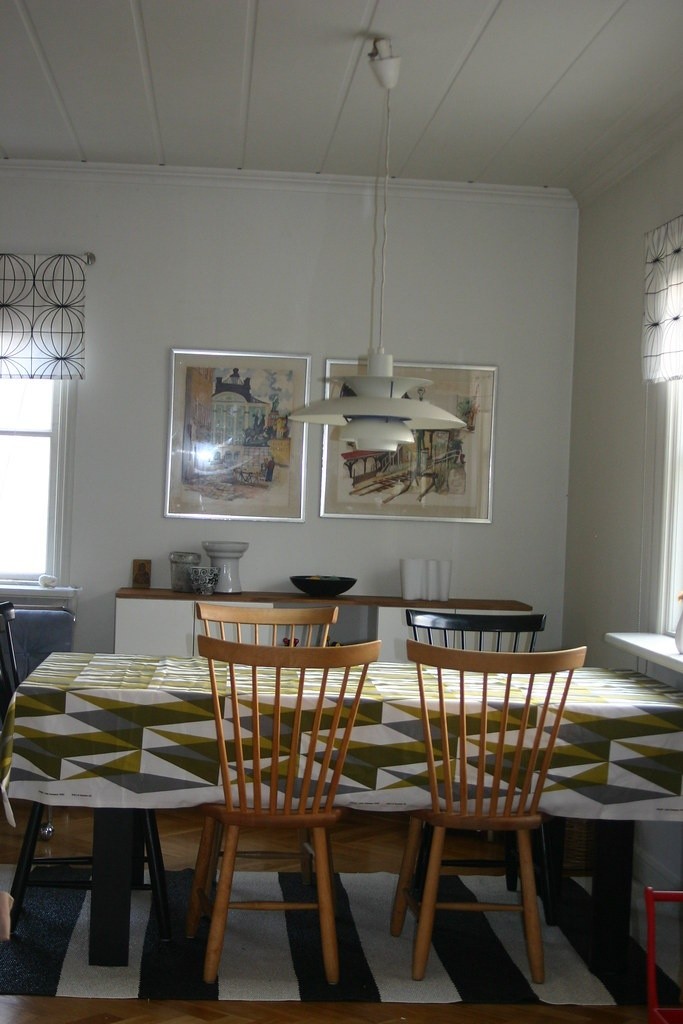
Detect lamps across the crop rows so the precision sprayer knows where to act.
[287,35,467,450]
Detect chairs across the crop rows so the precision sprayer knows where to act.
[391,637,588,983]
[406,609,546,890]
[193,601,339,648]
[185,635,382,987]
[0,602,170,940]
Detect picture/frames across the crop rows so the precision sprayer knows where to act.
[164,348,311,523]
[318,357,499,525]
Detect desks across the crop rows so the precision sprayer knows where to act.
[0,651,682,970]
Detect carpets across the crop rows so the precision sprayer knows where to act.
[0,859,683,1008]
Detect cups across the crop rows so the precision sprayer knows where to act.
[169,552,201,592]
[189,566,221,596]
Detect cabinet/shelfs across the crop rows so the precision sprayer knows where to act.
[114,587,533,664]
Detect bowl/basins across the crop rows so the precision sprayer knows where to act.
[290,575,357,597]
